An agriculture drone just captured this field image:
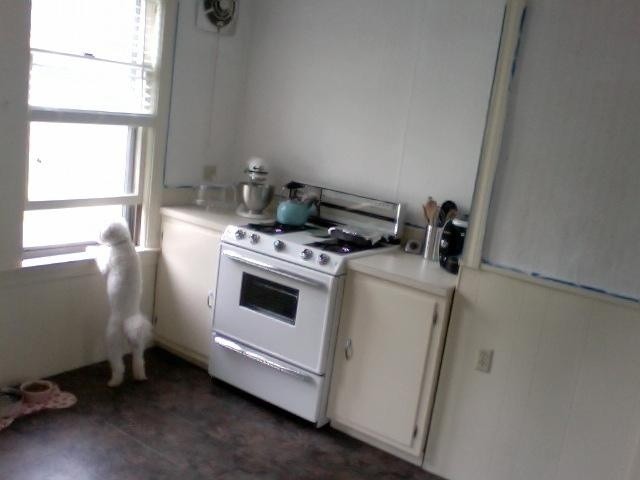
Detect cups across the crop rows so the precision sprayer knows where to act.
[239,182,275,214]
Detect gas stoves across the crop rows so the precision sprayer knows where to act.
[230,214,396,256]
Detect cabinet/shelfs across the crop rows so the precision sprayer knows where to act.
[327,261,452,470]
[150,210,220,371]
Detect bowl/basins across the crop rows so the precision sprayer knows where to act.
[0,380,53,418]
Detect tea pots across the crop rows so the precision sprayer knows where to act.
[277,188,316,225]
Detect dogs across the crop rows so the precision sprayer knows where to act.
[86,218,154,389]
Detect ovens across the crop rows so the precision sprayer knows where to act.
[207,224,343,423]
